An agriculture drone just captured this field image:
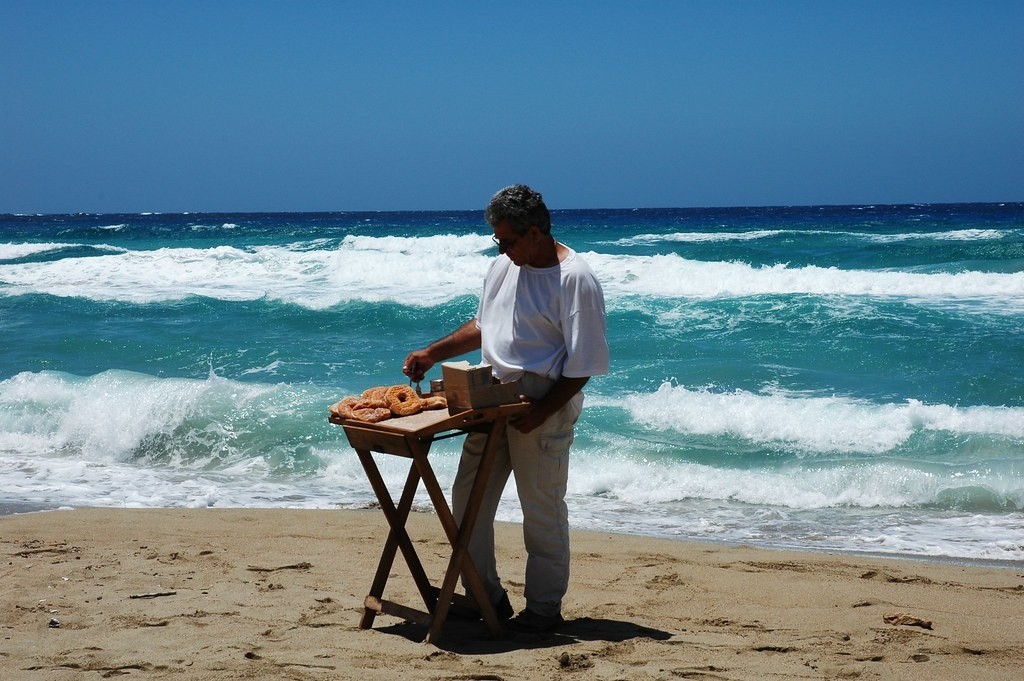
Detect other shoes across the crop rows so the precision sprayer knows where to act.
[506,607,565,632]
[467,588,514,622]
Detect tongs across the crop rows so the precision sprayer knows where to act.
[409,362,421,397]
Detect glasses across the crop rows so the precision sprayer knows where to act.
[492,234,520,251]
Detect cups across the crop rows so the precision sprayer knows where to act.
[430,379,445,392]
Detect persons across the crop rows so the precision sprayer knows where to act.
[403,185,609,641]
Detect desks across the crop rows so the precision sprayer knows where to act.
[324,390,531,645]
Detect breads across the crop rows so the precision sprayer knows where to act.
[330,384,447,422]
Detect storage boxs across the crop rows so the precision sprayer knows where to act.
[441,359,520,416]
